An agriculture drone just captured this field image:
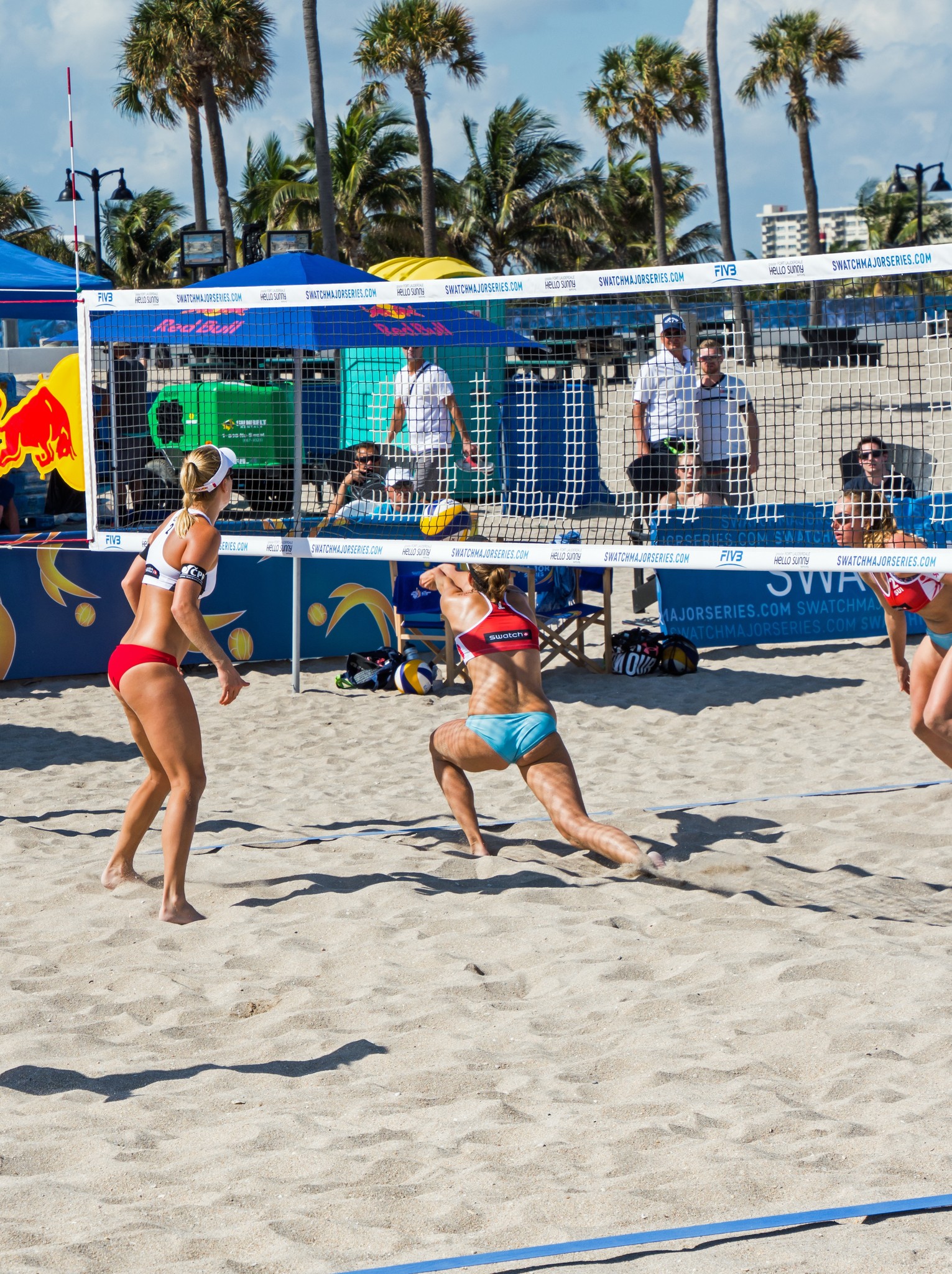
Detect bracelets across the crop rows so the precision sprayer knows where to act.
[138,543,151,560]
[178,562,207,584]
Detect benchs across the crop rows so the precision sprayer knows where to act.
[775,341,884,369]
[506,334,763,385]
[264,358,335,386]
[182,363,237,384]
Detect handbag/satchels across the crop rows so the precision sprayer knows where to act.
[339,646,405,690]
[607,629,663,676]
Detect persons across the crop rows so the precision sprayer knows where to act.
[830,434,952,768]
[0,477,20,535]
[95,441,254,926]
[632,314,762,516]
[25,320,74,347]
[327,346,481,517]
[417,537,666,875]
[94,342,151,527]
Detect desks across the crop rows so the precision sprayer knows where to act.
[801,326,862,368]
[532,324,623,360]
[626,323,655,363]
[701,319,753,357]
[537,340,576,385]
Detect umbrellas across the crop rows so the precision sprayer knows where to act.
[43,251,547,698]
[0,238,113,349]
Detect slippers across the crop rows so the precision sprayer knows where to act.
[622,616,662,628]
[456,448,495,477]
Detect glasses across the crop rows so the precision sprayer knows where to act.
[354,455,382,463]
[859,450,883,459]
[663,332,683,338]
[677,464,701,473]
[391,481,414,489]
[403,347,421,350]
[698,355,720,362]
[832,514,859,524]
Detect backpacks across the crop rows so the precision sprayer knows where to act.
[155,343,174,369]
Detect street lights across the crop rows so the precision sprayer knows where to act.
[55,167,136,278]
[885,161,952,320]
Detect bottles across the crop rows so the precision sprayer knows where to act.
[403,641,421,661]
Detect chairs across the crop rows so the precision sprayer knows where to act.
[324,441,417,498]
[838,444,938,499]
[625,452,708,546]
[291,484,335,513]
[333,499,383,518]
[19,468,87,534]
[217,492,256,521]
[388,560,614,687]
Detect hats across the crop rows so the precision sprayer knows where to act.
[385,467,415,486]
[660,313,687,332]
[103,341,131,354]
[179,444,237,492]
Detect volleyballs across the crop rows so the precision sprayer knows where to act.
[394,659,433,695]
[420,498,472,541]
[662,640,698,675]
